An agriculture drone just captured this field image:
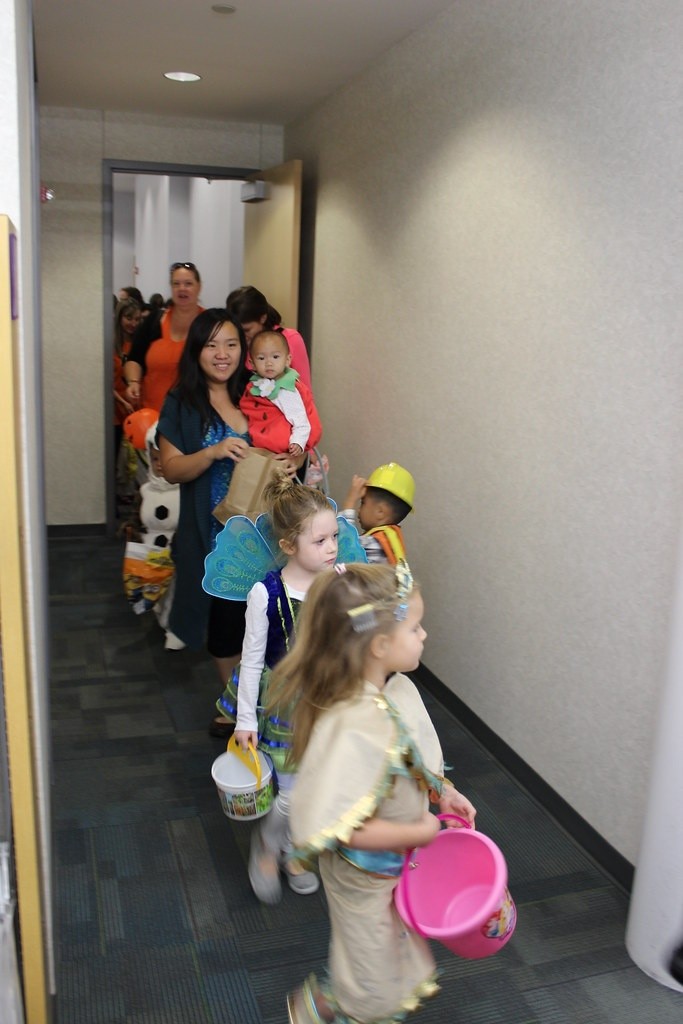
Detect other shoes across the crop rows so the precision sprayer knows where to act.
[280,849,320,895]
[210,711,238,737]
[248,824,282,905]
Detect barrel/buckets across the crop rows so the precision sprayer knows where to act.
[210,734,274,822]
[393,813,518,959]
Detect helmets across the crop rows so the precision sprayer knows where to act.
[364,463,416,514]
[123,408,160,449]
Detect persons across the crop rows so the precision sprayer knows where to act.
[155,309,323,676]
[113,262,311,615]
[269,564,476,1024]
[240,330,311,456]
[233,467,338,902]
[341,462,415,570]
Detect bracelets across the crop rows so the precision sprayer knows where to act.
[128,380,141,383]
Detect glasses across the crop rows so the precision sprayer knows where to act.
[170,263,194,271]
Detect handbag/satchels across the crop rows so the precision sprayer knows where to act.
[122,527,175,615]
